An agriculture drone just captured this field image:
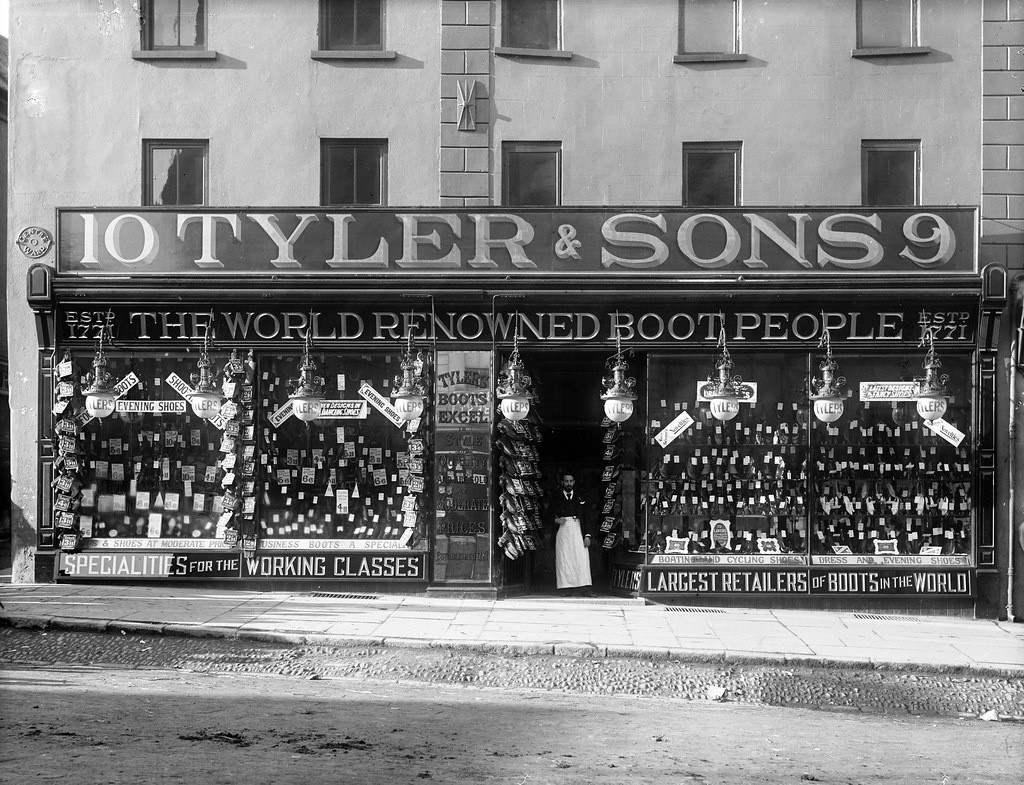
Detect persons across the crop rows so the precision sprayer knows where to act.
[548,470,597,596]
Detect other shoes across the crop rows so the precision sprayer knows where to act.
[583,589,597,597]
[561,589,573,597]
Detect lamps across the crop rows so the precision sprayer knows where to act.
[390,310,429,427]
[707,310,747,425]
[119,412,145,428]
[600,310,638,430]
[185,308,225,424]
[81,308,120,424]
[496,311,535,426]
[808,310,847,432]
[796,356,816,427]
[700,374,722,427]
[892,353,919,428]
[288,308,327,427]
[917,309,951,425]
[313,420,335,430]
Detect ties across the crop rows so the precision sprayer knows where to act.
[567,493,572,501]
